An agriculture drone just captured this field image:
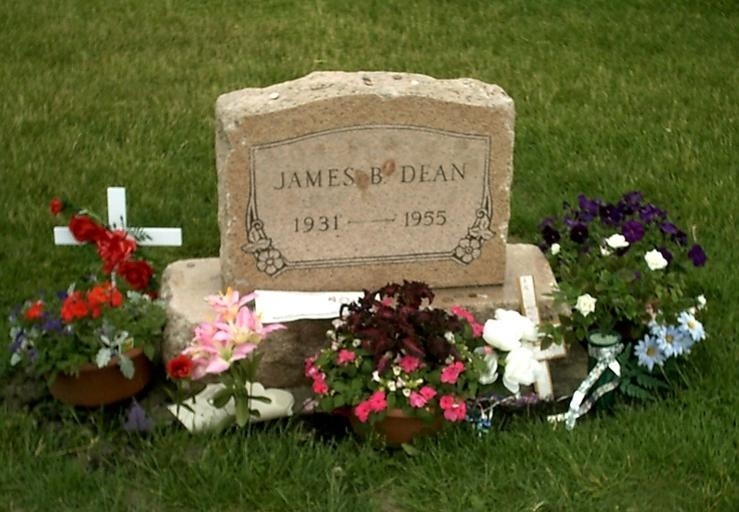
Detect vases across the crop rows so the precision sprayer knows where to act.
[574,291,662,355]
[351,403,438,448]
[46,347,145,406]
[581,329,623,418]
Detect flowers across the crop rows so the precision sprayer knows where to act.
[302,282,501,425]
[241,217,286,281]
[454,203,496,267]
[542,189,716,372]
[7,197,167,388]
[534,234,670,353]
